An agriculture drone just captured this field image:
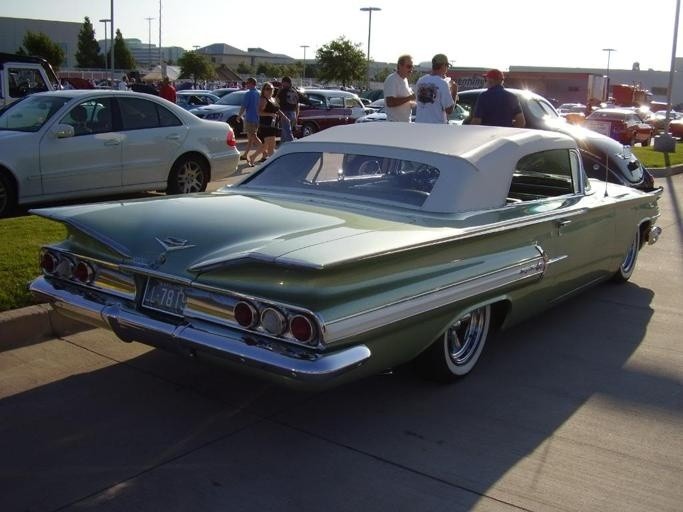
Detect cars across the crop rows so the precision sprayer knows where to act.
[335,86,663,252]
[555,96,683,149]
[0,90,241,217]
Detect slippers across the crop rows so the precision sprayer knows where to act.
[239,155,267,167]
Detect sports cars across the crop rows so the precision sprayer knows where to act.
[25,117,664,391]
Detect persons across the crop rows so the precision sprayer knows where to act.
[194,78,299,167]
[584,98,594,117]
[416,54,458,123]
[17,75,176,105]
[383,55,416,121]
[470,69,526,128]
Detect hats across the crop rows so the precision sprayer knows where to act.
[481,68,504,81]
[431,53,449,65]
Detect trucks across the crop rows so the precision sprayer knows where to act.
[422,68,674,117]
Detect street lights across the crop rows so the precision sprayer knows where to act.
[98,18,111,70]
[144,17,154,63]
[602,47,616,77]
[299,44,310,79]
[192,46,200,51]
[359,7,381,86]
